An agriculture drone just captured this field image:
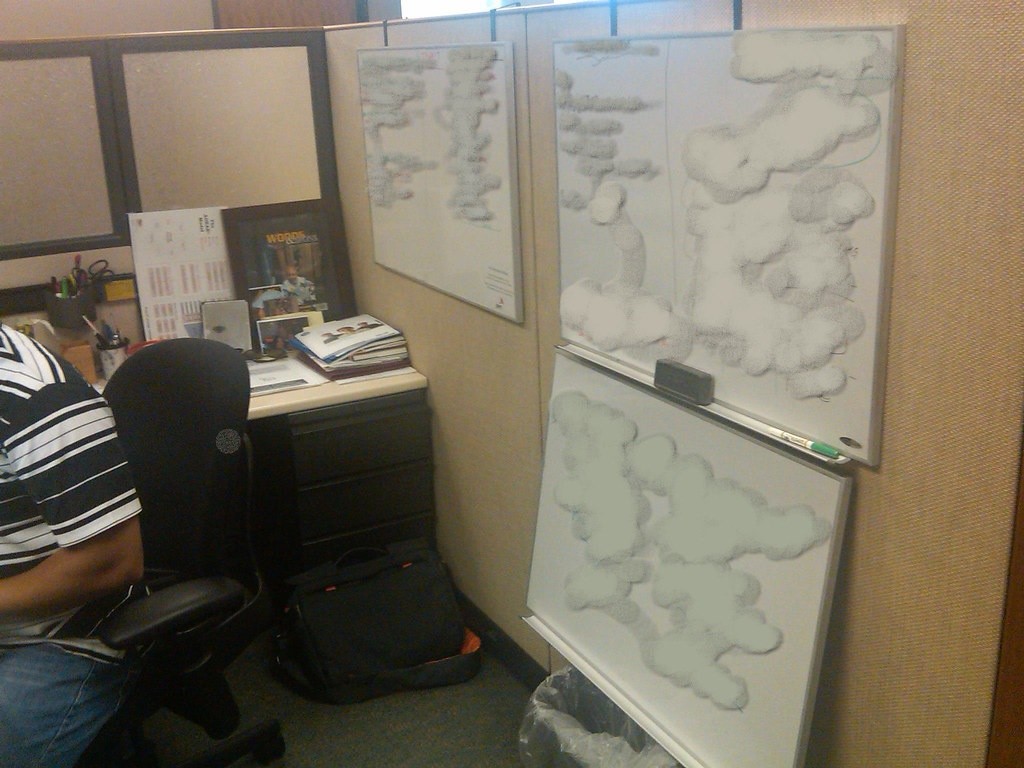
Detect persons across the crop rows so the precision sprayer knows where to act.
[338,327,369,339]
[254,267,317,318]
[0,323,144,768]
[322,333,343,344]
[357,322,384,329]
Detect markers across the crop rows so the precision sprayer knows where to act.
[767,427,840,459]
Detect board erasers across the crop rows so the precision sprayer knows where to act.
[653,358,713,405]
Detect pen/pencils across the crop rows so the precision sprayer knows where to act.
[81,315,121,348]
[50,252,92,298]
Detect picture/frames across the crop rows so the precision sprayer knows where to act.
[220,198,353,357]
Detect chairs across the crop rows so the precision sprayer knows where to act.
[73,339,287,768]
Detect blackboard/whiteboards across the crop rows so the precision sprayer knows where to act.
[550,23,906,471]
[355,39,524,325]
[517,347,854,768]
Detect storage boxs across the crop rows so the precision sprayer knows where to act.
[61,339,97,382]
[26,288,97,326]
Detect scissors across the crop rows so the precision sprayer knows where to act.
[88,259,114,285]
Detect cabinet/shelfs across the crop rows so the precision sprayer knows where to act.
[88,357,438,567]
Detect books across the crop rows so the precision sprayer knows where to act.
[290,314,417,384]
